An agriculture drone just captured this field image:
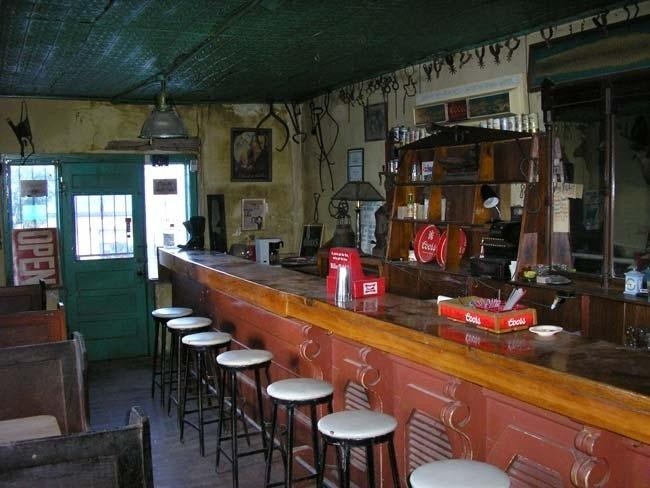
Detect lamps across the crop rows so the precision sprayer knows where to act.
[330,181,385,248]
[136,74,187,138]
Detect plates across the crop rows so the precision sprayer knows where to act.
[529,325,563,337]
[552,278,572,285]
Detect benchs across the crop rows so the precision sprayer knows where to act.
[0,279,154,488]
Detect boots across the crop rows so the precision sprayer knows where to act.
[177,216,205,249]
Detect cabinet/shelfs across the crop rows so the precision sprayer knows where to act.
[506,67,650,344]
[382,123,545,300]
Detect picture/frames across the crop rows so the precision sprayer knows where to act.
[299,223,324,257]
[207,194,227,251]
[347,148,364,183]
[230,128,273,182]
[526,15,650,93]
[242,198,266,230]
[363,102,389,142]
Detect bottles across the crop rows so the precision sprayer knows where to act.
[393,125,441,148]
[407,193,423,220]
[479,113,539,133]
[411,161,422,181]
[448,101,468,120]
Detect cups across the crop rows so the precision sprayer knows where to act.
[335,267,352,302]
[625,326,650,349]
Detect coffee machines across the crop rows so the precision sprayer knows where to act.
[255,238,284,268]
